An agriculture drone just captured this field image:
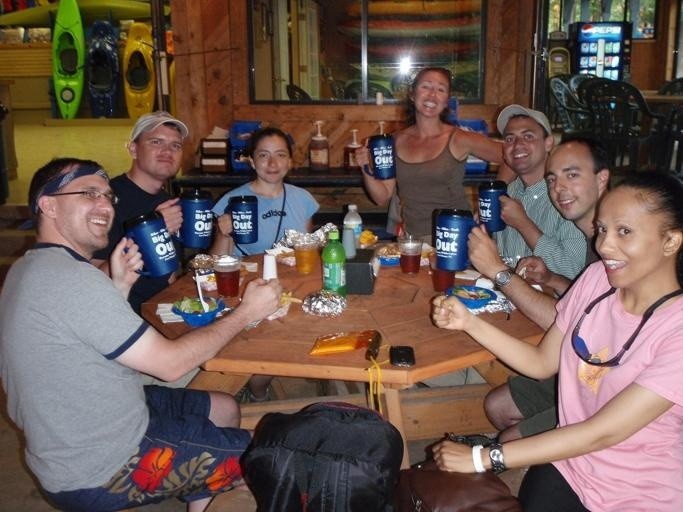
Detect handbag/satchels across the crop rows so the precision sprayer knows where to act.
[396,459,524,512]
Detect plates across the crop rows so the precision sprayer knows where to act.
[378,254,399,267]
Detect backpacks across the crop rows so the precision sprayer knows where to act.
[238,402,404,512]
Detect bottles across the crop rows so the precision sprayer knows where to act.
[344,205,361,249]
[344,130,363,173]
[309,120,330,171]
[322,231,347,296]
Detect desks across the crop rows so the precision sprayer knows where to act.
[172,164,499,198]
[138,237,565,475]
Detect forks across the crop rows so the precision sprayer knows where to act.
[194,272,210,312]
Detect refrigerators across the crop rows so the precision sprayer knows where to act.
[568,21,633,85]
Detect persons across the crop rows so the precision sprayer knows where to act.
[108,112,202,306]
[468,135,631,446]
[357,67,516,246]
[3,156,285,510]
[476,104,587,280]
[214,127,321,402]
[432,168,683,512]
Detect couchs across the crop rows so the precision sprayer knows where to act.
[200,456,524,512]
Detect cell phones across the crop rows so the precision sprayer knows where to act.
[389,346,416,368]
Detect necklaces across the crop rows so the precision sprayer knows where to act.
[571,286,682,367]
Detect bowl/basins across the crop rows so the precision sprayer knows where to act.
[170,297,224,326]
[447,286,497,308]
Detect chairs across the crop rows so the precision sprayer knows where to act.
[391,73,413,91]
[450,78,478,97]
[660,77,683,173]
[577,77,610,103]
[548,78,594,138]
[285,84,312,100]
[344,81,394,99]
[330,80,346,99]
[570,73,600,92]
[587,80,667,165]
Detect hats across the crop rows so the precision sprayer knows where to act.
[131,111,189,141]
[497,104,552,136]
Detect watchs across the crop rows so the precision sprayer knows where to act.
[491,267,517,290]
[488,442,507,473]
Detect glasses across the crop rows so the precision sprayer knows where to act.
[36,190,119,218]
[572,311,626,367]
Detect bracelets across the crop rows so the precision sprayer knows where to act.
[473,442,484,471]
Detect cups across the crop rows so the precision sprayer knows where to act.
[173,189,212,250]
[229,195,258,243]
[121,211,181,278]
[400,238,423,274]
[294,243,316,273]
[364,133,396,179]
[428,250,455,291]
[214,262,240,297]
[436,209,480,271]
[479,180,510,233]
[342,228,357,259]
